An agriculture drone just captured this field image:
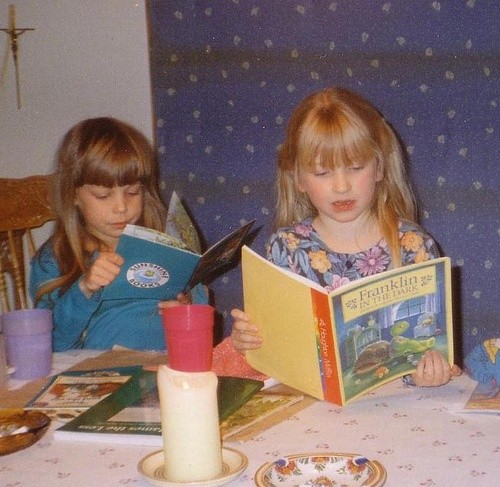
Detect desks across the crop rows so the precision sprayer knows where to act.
[0,350,500,487]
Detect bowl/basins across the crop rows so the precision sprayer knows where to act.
[137,446,248,487]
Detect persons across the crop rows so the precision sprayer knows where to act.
[231,86,461,388]
[28,117,209,354]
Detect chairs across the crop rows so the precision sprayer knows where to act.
[0,174,55,311]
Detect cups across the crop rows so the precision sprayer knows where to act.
[165,305,214,372]
[0,309,53,379]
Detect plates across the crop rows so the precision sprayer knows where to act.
[0,410,50,456]
[255,452,387,487]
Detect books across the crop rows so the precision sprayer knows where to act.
[241,245,453,407]
[98,191,256,303]
[23,364,304,446]
